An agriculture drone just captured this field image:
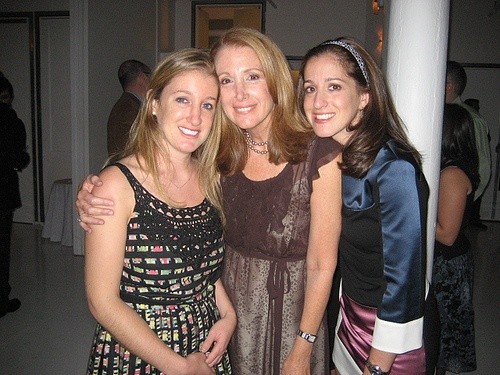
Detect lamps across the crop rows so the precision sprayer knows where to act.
[371,0,385,14]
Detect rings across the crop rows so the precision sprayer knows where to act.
[77,212,87,222]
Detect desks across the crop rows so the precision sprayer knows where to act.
[41,178,74,247]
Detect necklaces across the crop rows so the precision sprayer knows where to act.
[244,132,269,155]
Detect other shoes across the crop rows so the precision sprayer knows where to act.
[0,298,21,318]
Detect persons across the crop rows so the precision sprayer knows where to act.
[295,37,442,374]
[432,60,492,374]
[107,59,153,163]
[0,71,31,318]
[76,26,345,375]
[84,50,238,375]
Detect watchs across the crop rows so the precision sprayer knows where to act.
[296,330,317,343]
[365,358,391,375]
